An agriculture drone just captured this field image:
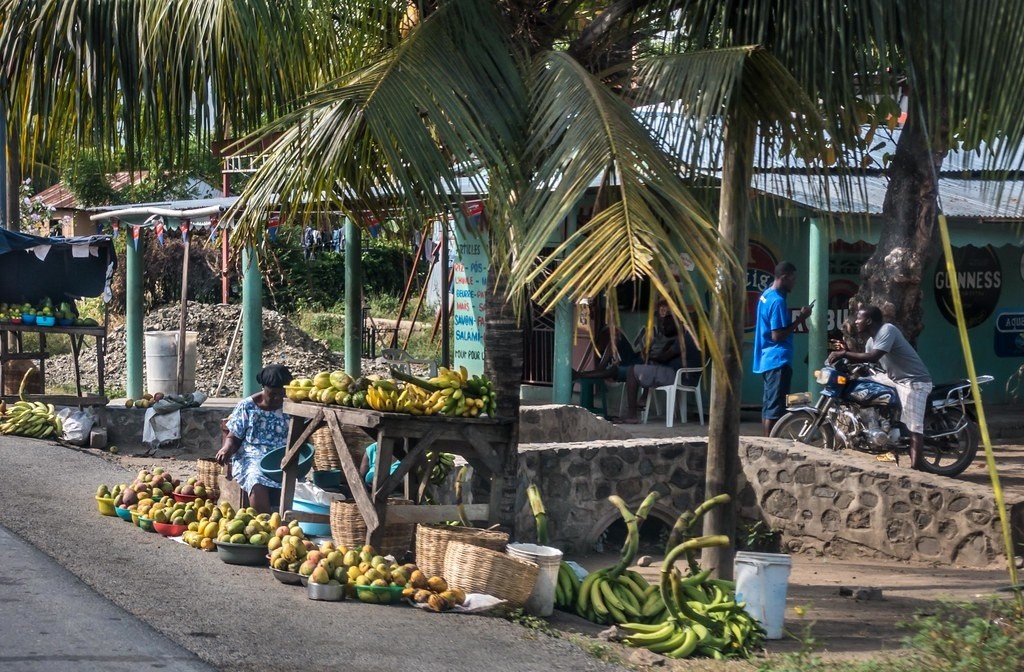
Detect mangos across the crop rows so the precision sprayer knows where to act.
[125,393,164,408]
[55,311,99,326]
[110,446,119,453]
[288,370,398,407]
[96,468,467,612]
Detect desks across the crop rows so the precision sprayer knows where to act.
[0,323,108,429]
[278,397,517,559]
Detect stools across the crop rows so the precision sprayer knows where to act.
[571,376,613,423]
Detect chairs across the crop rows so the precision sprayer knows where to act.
[618,357,712,427]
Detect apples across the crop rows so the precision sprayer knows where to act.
[0,302,53,319]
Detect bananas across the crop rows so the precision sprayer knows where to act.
[0,400,62,439]
[554,560,768,662]
[366,366,496,419]
[426,452,456,485]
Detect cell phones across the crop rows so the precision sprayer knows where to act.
[810,300,816,306]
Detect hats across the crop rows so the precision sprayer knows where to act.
[256,365,294,387]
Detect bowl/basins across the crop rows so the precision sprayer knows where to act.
[306,582,344,601]
[149,498,160,502]
[171,491,198,502]
[129,511,141,527]
[138,516,154,531]
[355,584,405,603]
[212,538,272,565]
[259,442,315,483]
[151,522,187,536]
[94,495,116,515]
[115,507,131,521]
[0,318,22,324]
[269,565,309,586]
[21,315,36,324]
[312,470,342,492]
[57,319,74,325]
[36,316,55,326]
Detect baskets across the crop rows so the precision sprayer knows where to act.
[197,458,224,500]
[330,500,415,562]
[444,541,539,607]
[309,426,378,470]
[416,523,509,579]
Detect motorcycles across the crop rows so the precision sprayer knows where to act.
[768,358,996,478]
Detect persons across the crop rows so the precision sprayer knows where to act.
[358,442,405,489]
[752,262,813,436]
[215,364,293,514]
[573,311,709,424]
[828,306,932,470]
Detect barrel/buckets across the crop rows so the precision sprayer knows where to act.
[144,331,199,397]
[733,551,791,639]
[292,497,330,536]
[506,543,564,615]
